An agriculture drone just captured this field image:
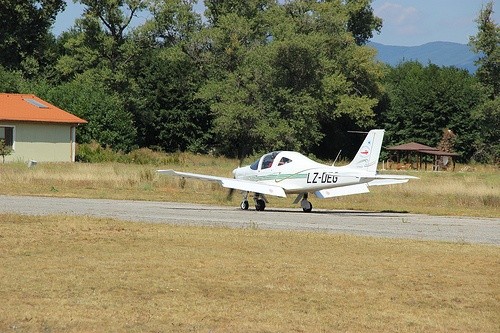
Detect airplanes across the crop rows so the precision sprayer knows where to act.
[154,128,422,213]
